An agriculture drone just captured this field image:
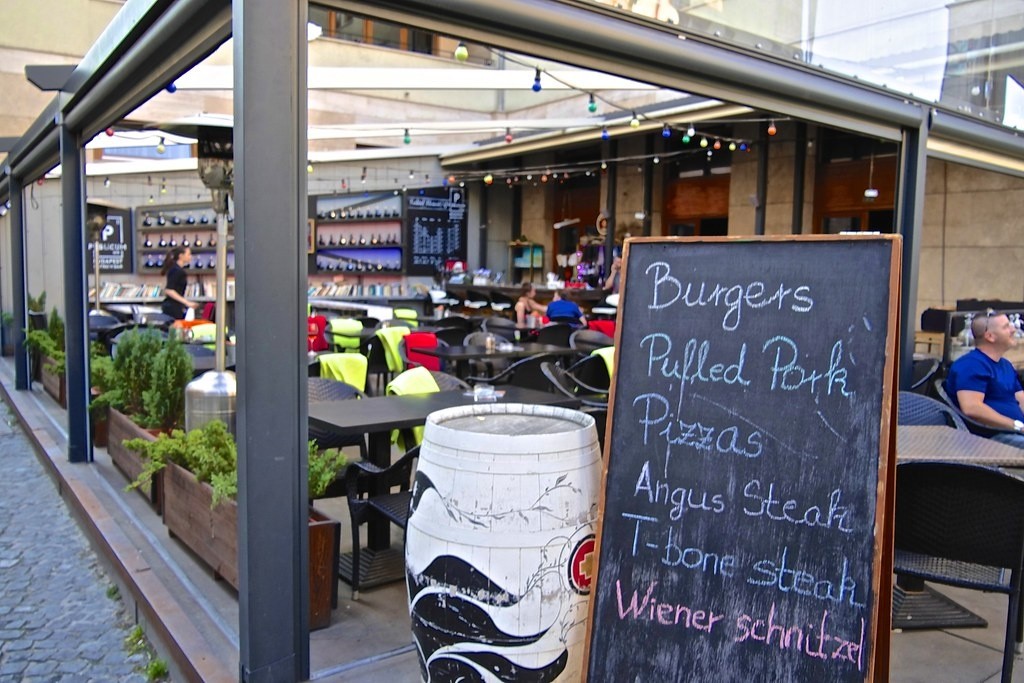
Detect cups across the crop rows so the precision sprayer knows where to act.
[486,336,495,354]
[474,385,496,404]
[134,313,149,324]
[527,316,536,328]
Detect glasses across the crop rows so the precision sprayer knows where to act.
[985,307,995,331]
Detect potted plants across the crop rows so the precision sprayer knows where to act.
[0,289,345,632]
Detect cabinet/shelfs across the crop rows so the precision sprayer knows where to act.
[136,201,236,276]
[508,242,545,284]
[308,189,408,276]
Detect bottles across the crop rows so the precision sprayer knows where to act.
[185,211,233,224]
[320,207,398,219]
[318,233,399,245]
[169,234,177,247]
[182,234,190,248]
[158,212,165,225]
[537,312,543,328]
[956,320,975,347]
[195,255,203,269]
[208,257,215,269]
[172,212,180,224]
[194,234,202,247]
[159,232,168,247]
[156,255,164,266]
[144,233,152,247]
[1013,321,1023,339]
[208,233,216,246]
[143,212,152,226]
[317,258,401,271]
[146,254,154,267]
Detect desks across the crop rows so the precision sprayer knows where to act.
[309,384,581,592]
[325,323,470,397]
[90,295,430,329]
[915,332,945,356]
[111,337,171,347]
[108,304,161,321]
[495,321,581,341]
[89,321,164,342]
[193,350,337,379]
[410,343,571,389]
[891,425,1024,630]
[407,312,483,325]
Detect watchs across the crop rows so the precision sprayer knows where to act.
[1014,419,1023,432]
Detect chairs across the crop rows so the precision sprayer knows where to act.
[88,290,617,599]
[898,359,1024,683]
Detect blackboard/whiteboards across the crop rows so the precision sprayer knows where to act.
[581,233,903,683]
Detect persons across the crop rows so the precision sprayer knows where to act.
[545,288,588,328]
[159,245,199,320]
[601,253,622,295]
[512,281,548,326]
[944,310,1024,449]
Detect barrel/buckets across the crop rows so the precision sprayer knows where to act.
[405,403,601,683]
